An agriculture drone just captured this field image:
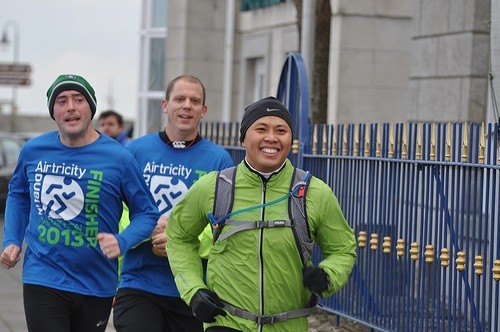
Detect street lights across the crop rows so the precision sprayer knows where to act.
[1,18,20,133]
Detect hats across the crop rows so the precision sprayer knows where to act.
[240,96,294,144]
[47,74,97,121]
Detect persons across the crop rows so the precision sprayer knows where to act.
[165,96,358,332]
[97,110,128,150]
[113,74,235,332]
[0,74,161,332]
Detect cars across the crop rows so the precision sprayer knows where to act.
[0,137,26,201]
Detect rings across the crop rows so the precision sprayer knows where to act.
[106,248,110,254]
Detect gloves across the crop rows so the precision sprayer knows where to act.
[190,289,227,323]
[303,267,328,294]
